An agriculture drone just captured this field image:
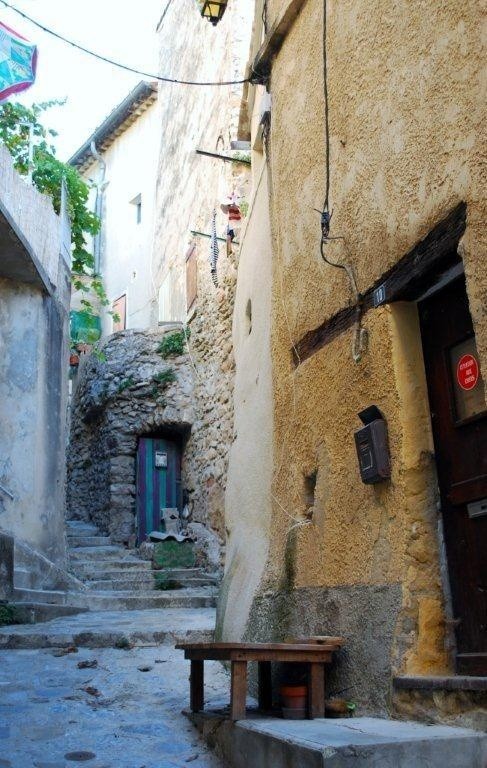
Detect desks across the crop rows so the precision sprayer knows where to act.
[174,641,341,722]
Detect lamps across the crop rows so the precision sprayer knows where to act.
[195,0,229,27]
